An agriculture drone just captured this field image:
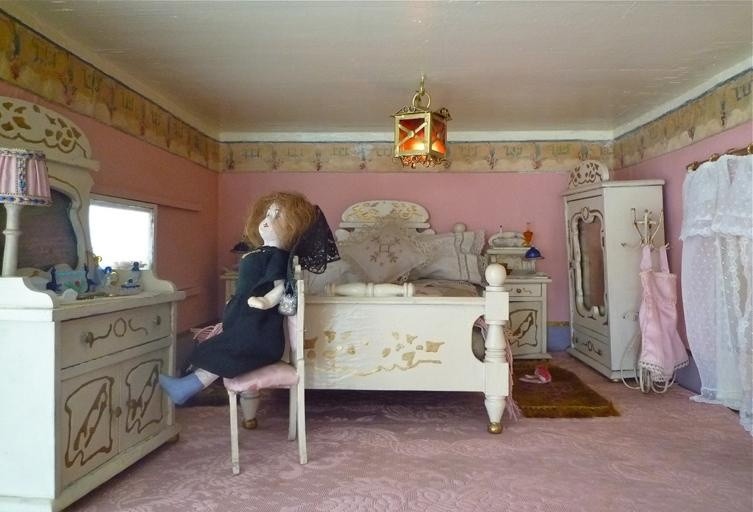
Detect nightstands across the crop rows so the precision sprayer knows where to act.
[220,270,239,312]
[481,228,552,364]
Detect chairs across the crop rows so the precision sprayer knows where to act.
[220,254,307,478]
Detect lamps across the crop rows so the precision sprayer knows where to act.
[0,144,53,279]
[386,72,454,172]
[230,239,250,265]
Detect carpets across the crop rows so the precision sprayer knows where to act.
[505,357,624,423]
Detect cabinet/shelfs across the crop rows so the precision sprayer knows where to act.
[0,267,188,510]
[559,158,668,383]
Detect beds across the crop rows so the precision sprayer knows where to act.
[270,199,512,435]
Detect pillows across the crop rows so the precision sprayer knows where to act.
[330,207,436,288]
[294,224,487,301]
